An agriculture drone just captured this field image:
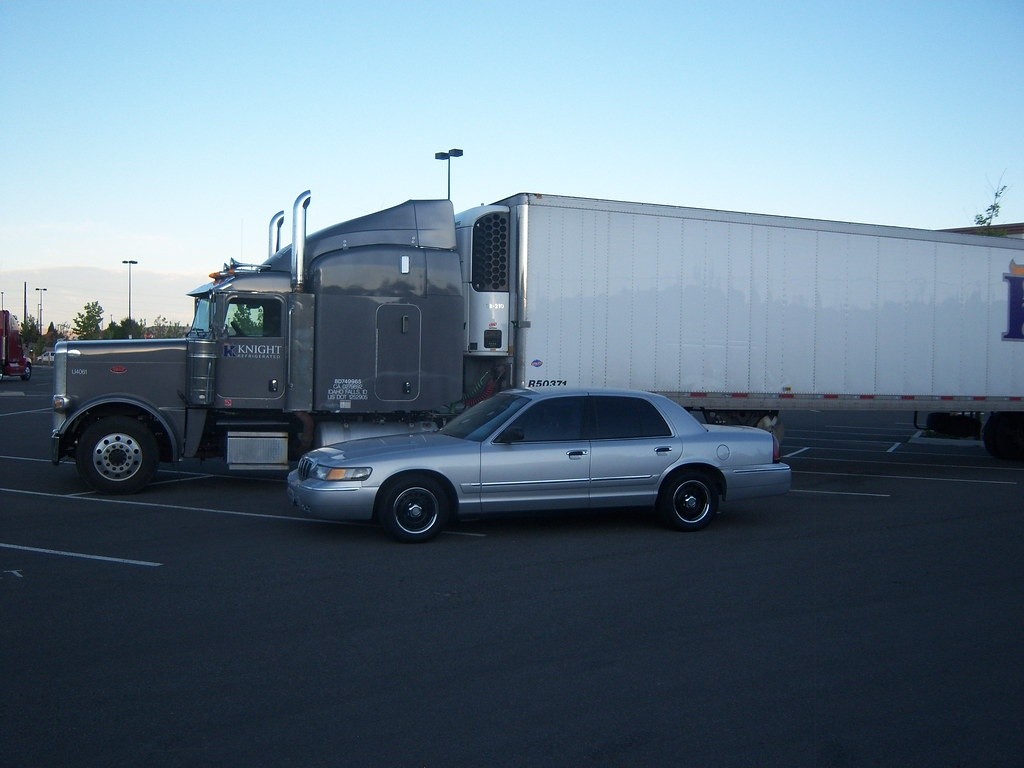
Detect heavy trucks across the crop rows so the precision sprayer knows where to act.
[42,186,1023,474]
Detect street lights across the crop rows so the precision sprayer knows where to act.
[122,260,137,320]
[35,288,47,336]
[435,148,465,199]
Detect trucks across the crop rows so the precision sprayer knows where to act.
[0,310,33,383]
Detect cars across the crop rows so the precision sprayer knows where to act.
[36,350,54,363]
[285,384,793,534]
